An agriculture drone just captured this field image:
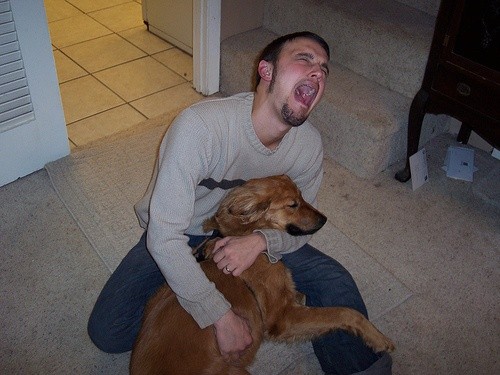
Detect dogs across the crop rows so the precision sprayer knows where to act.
[129,174,397,375]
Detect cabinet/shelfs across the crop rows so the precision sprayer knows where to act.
[395,0,500,183]
